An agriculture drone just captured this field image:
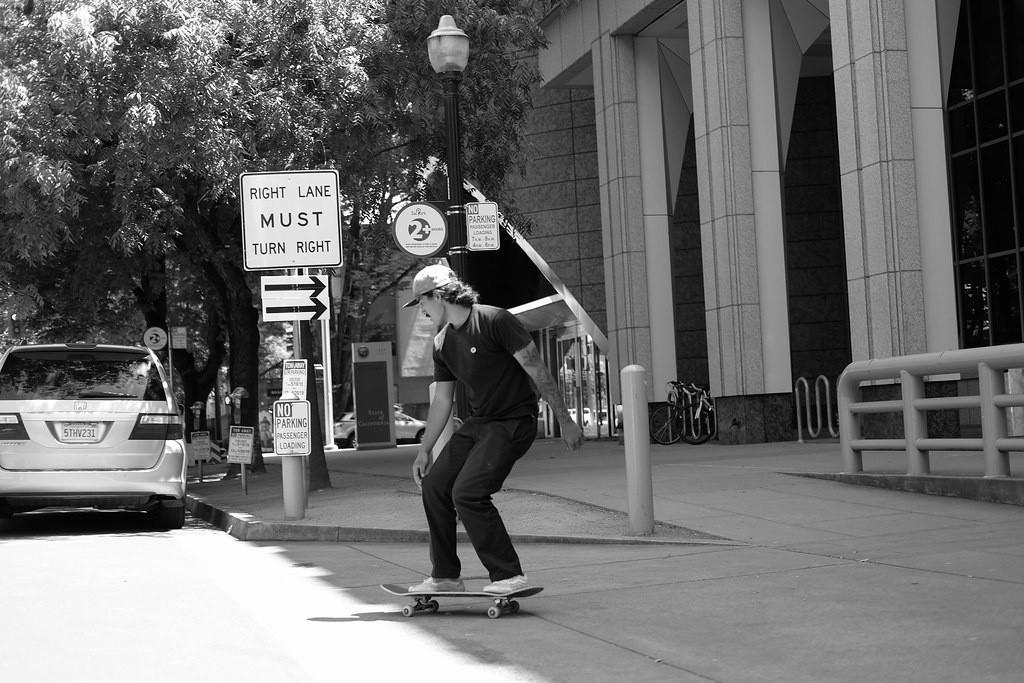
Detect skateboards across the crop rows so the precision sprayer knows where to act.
[380,583,545,619]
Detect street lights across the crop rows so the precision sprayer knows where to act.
[426,13,474,288]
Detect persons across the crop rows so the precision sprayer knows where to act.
[402,265,584,594]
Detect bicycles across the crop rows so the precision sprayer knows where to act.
[649,379,716,445]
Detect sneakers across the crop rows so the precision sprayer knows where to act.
[483,573,528,594]
[409,577,465,592]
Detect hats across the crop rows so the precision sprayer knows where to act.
[402,264,457,307]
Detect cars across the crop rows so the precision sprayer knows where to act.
[334,405,427,449]
[568,408,610,428]
[0,340,188,530]
